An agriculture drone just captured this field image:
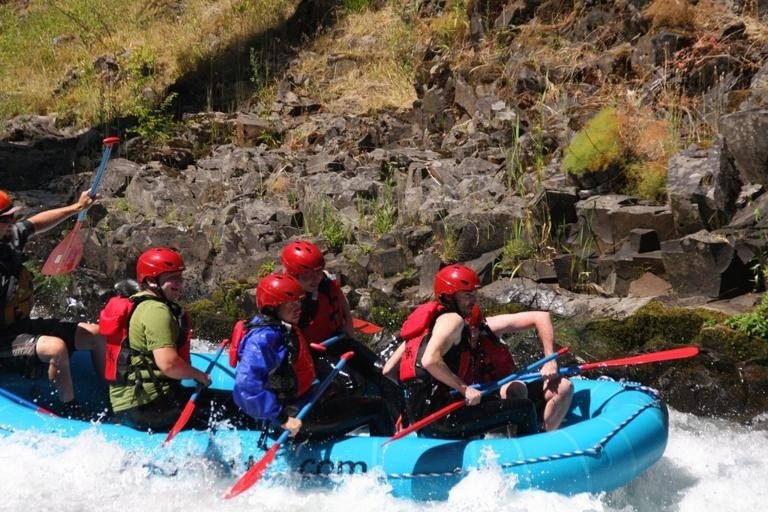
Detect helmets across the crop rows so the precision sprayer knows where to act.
[435,265,482,316]
[282,240,326,295]
[0,190,13,244]
[256,272,307,323]
[136,247,187,304]
[466,304,481,328]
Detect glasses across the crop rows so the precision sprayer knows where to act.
[1,217,16,224]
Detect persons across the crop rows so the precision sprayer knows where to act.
[278,241,410,431]
[380,310,575,432]
[229,273,395,446]
[98,247,213,433]
[0,188,103,422]
[399,264,539,440]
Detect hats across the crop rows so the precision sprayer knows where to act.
[0,206,25,219]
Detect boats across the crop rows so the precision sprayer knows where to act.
[0,350,668,509]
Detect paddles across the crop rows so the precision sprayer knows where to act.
[390,345,570,445]
[309,316,386,354]
[223,350,356,502]
[160,337,230,450]
[41,136,121,278]
[448,344,700,396]
